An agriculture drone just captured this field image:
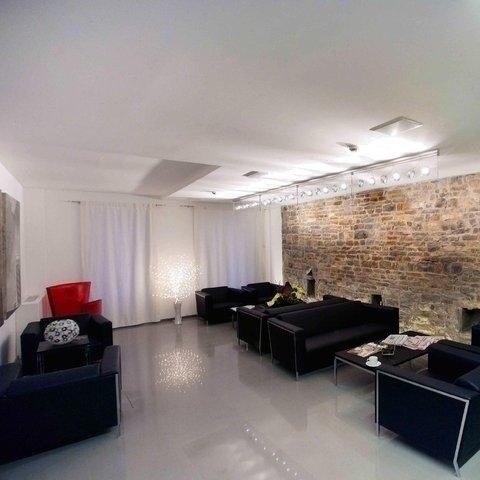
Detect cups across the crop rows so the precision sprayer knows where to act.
[367,356,379,364]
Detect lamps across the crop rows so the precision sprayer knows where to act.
[148,256,201,324]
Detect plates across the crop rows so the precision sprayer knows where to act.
[366,361,381,367]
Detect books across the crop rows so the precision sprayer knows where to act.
[346,342,382,358]
[384,334,408,346]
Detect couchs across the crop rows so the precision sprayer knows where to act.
[237,295,399,380]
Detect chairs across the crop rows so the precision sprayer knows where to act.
[376,325,480,477]
[195,282,276,326]
[0,314,121,466]
